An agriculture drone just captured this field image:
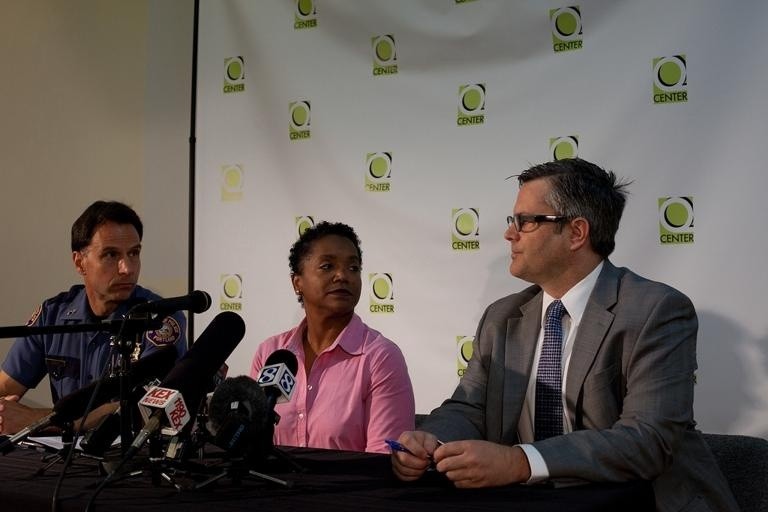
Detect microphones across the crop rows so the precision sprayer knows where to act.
[125,289,211,320]
[130,309,245,457]
[258,349,297,431]
[207,374,269,463]
[162,363,230,468]
[80,374,162,457]
[52,342,180,425]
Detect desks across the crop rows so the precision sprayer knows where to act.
[1,444,657,510]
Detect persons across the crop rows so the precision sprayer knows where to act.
[388,152,743,511]
[250,221,418,455]
[0,200,189,438]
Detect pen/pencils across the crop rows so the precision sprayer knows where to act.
[384,439,433,461]
[249,470,288,486]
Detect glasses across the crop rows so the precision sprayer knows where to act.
[506,212,575,233]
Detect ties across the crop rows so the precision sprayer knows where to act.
[533,298,569,444]
